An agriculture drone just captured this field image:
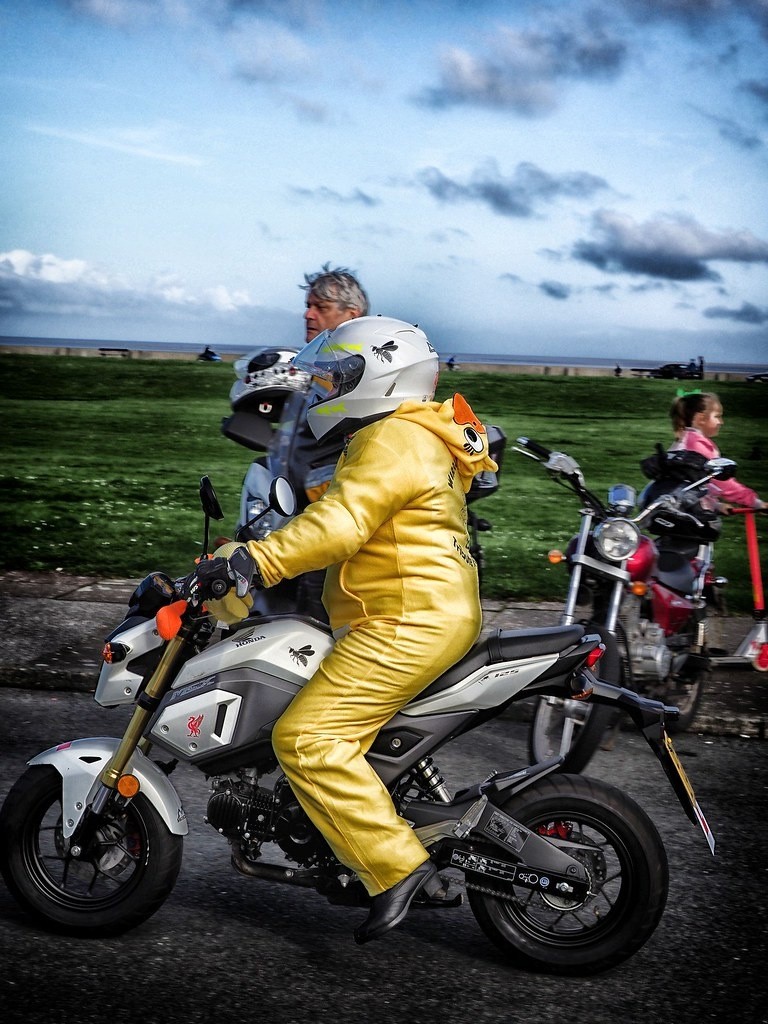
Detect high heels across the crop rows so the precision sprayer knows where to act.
[353,859,443,946]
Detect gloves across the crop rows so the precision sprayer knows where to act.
[174,546,264,613]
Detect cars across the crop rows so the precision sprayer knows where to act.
[652,364,702,379]
[746,371,768,384]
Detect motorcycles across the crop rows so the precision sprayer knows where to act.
[512,433,739,775]
[1,474,671,979]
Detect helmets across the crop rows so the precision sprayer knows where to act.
[291,315,440,448]
[228,345,312,414]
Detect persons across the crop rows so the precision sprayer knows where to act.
[667,389,768,596]
[183,316,500,945]
[274,261,370,626]
